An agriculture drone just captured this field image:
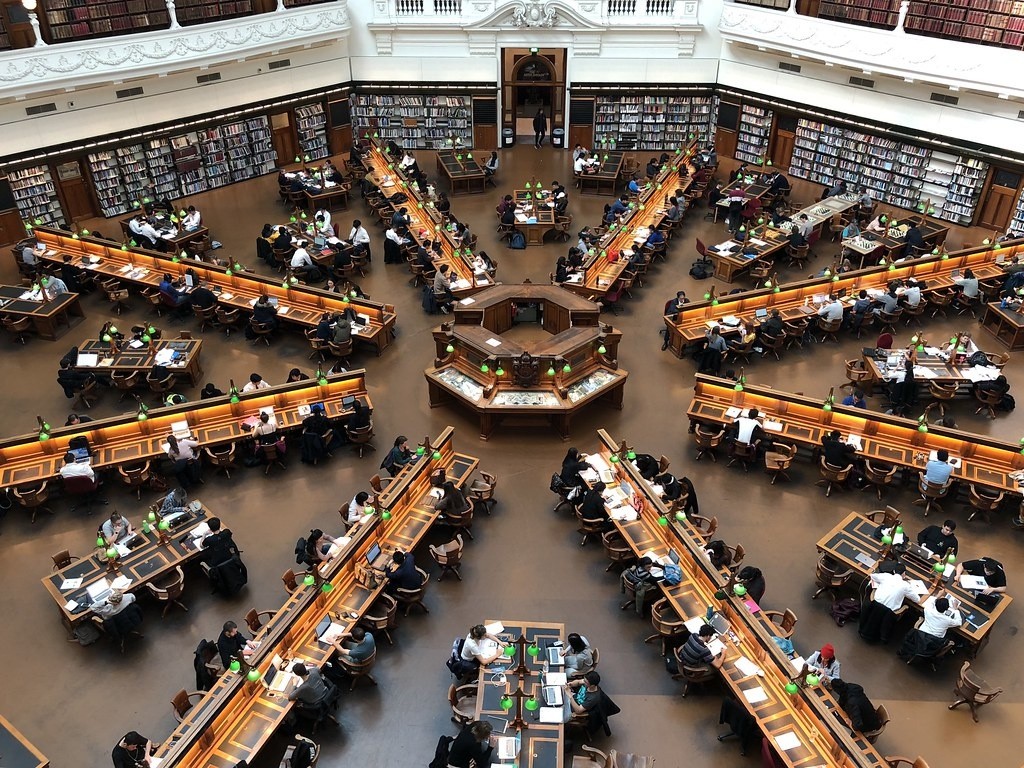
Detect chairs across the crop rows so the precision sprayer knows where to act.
[1,139,1023,768]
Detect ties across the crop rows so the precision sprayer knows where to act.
[353,229,358,238]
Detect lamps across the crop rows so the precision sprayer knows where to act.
[21,127,574,729]
[587,131,1024,700]
[21,0,37,15]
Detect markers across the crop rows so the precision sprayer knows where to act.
[306,664,313,666]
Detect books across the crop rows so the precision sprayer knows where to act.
[0,12,11,48]
[595,96,719,150]
[348,95,467,148]
[736,105,986,224]
[295,104,328,160]
[39,0,333,41]
[7,168,60,234]
[88,115,277,217]
[735,0,1024,50]
[1005,187,1024,236]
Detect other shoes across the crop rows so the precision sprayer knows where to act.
[538,142,543,148]
[534,143,539,149]
[1012,518,1023,527]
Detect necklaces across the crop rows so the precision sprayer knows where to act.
[126,749,138,761]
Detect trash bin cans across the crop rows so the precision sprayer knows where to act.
[553,128,564,147]
[502,128,514,147]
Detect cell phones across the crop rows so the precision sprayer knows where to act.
[350,612,359,619]
[81,603,91,607]
[267,693,275,697]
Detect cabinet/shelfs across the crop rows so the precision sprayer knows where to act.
[1002,187,1024,238]
[735,107,780,167]
[350,94,473,151]
[6,163,70,244]
[787,118,993,220]
[294,101,328,162]
[737,1,1023,48]
[88,114,281,219]
[0,0,318,51]
[596,90,710,152]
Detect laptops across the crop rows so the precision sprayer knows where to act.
[80,256,90,267]
[213,285,223,295]
[342,395,355,409]
[141,207,188,231]
[310,401,327,416]
[855,542,999,618]
[657,547,681,566]
[709,612,732,636]
[498,635,516,660]
[48,288,63,298]
[813,294,826,308]
[540,679,564,706]
[498,729,522,759]
[838,287,850,301]
[285,143,461,283]
[243,543,393,691]
[36,243,47,252]
[267,296,278,309]
[67,447,91,465]
[544,640,565,665]
[85,510,192,602]
[955,354,967,359]
[755,307,769,322]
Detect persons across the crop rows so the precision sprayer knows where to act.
[713,163,925,255]
[719,369,1024,525]
[350,141,493,314]
[555,140,716,311]
[498,180,568,235]
[447,625,602,768]
[23,224,368,489]
[889,332,1009,405]
[113,434,469,768]
[128,195,202,252]
[533,107,548,149]
[94,479,232,641]
[260,161,370,278]
[868,519,1007,659]
[562,447,879,738]
[484,151,499,175]
[664,231,1024,375]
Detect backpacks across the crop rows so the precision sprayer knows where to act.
[542,228,565,242]
[508,228,527,249]
[689,268,709,279]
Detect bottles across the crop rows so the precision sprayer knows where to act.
[287,648,293,660]
[706,604,713,619]
[1000,298,1007,310]
[142,520,150,533]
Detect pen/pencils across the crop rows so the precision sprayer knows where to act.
[534,717,539,720]
[961,610,965,616]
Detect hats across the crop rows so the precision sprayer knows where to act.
[173,487,188,500]
[164,273,176,280]
[821,644,835,658]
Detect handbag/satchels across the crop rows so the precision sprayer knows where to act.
[571,489,584,510]
[566,487,581,500]
[628,492,644,520]
[240,415,260,431]
[607,245,619,262]
[541,204,552,211]
[742,245,759,256]
[679,163,689,177]
[430,467,446,487]
[431,236,442,251]
[662,563,681,585]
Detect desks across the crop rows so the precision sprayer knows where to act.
[573,423,900,766]
[19,216,396,358]
[422,282,633,441]
[790,193,863,249]
[255,223,365,282]
[0,287,88,345]
[44,500,244,646]
[979,298,1022,352]
[573,149,627,197]
[0,368,377,520]
[77,339,203,387]
[435,146,486,195]
[142,427,488,767]
[709,224,794,283]
[861,217,952,264]
[816,508,1012,666]
[662,238,1021,367]
[712,171,775,223]
[838,235,884,272]
[564,135,715,314]
[686,368,1022,523]
[119,210,215,258]
[860,342,1000,403]
[351,146,499,301]
[477,620,570,767]
[510,186,554,248]
[291,166,344,211]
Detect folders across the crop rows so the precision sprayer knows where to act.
[744,600,760,614]
[965,609,990,630]
